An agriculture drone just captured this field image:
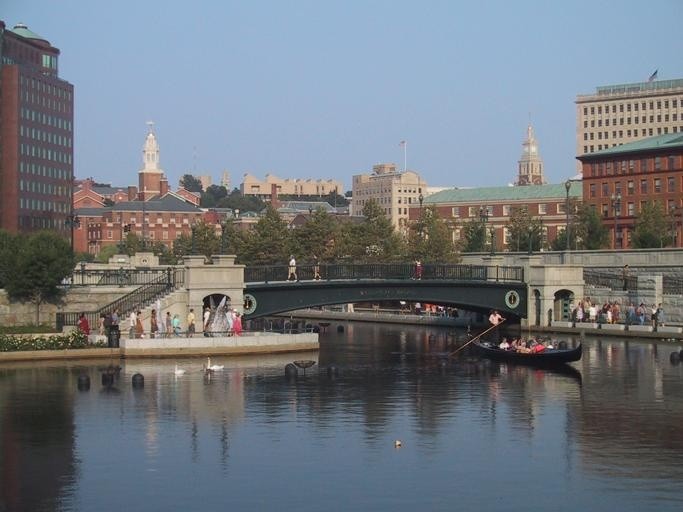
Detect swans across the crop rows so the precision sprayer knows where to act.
[207,357,225,371]
[173,361,186,375]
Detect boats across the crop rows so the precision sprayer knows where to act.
[467,324,582,365]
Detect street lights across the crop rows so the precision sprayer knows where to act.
[191,222,198,255]
[526,213,544,256]
[479,206,496,256]
[564,180,571,250]
[64,214,80,287]
[611,192,622,248]
[220,220,227,255]
[419,195,424,234]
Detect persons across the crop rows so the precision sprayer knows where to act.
[569,297,665,327]
[415,260,423,280]
[77,312,89,335]
[622,265,630,291]
[102,307,243,338]
[312,254,321,281]
[342,300,459,320]
[489,310,558,353]
[286,255,297,282]
